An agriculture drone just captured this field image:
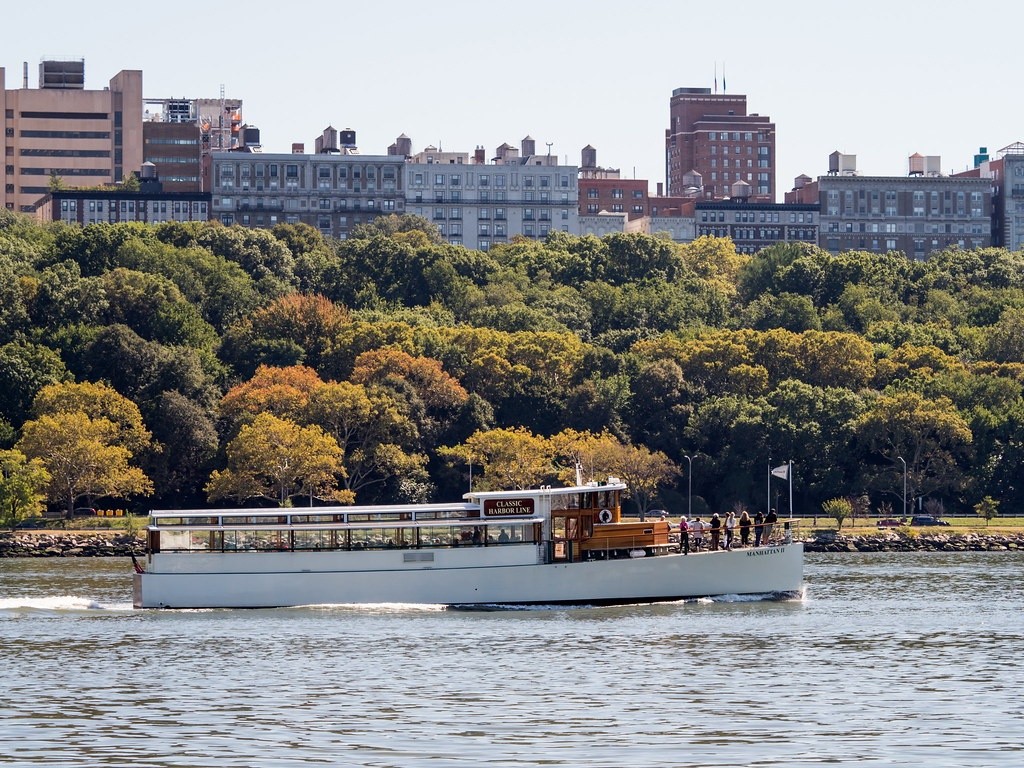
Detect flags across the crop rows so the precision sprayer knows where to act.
[771,465,788,480]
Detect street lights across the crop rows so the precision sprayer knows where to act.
[767,457,772,513]
[898,457,906,518]
[685,455,698,520]
[789,459,795,521]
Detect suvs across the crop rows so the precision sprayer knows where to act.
[877,518,908,526]
[910,516,951,526]
[74,508,96,516]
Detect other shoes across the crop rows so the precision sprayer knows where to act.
[742,545,748,548]
[726,547,733,551]
[685,552,687,555]
[710,548,719,551]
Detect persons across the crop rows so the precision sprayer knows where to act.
[721,512,730,550]
[709,513,721,551]
[753,511,764,547]
[739,511,751,549]
[762,509,777,544]
[679,516,689,555]
[725,512,736,551]
[154,525,537,551]
[657,515,666,522]
[692,517,704,553]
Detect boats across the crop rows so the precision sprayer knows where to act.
[132,482,805,612]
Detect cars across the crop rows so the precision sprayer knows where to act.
[648,509,668,514]
